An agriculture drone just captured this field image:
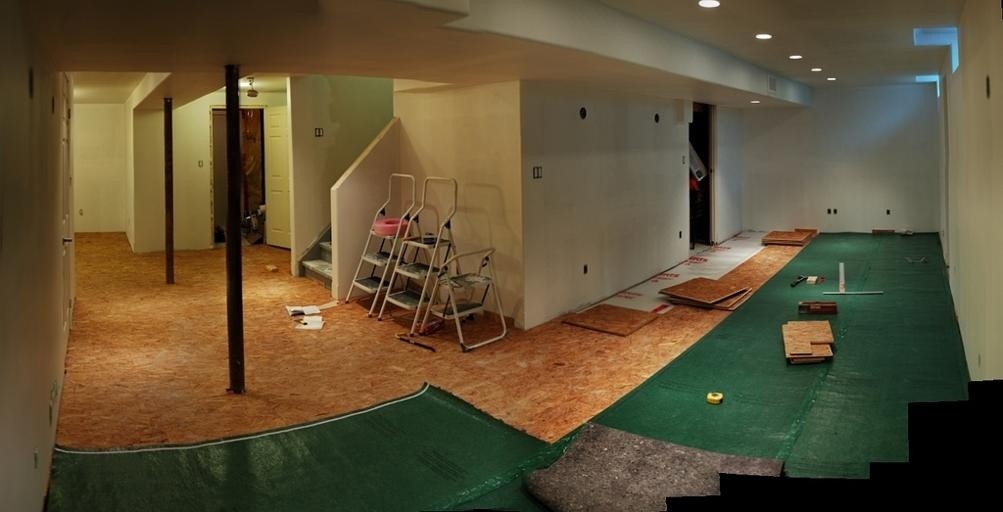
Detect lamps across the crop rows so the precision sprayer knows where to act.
[247,77,258,97]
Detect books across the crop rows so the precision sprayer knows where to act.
[296,316,326,330]
[287,304,320,316]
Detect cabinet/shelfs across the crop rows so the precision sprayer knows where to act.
[346,173,508,353]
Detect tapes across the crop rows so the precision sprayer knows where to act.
[707,393,723,404]
